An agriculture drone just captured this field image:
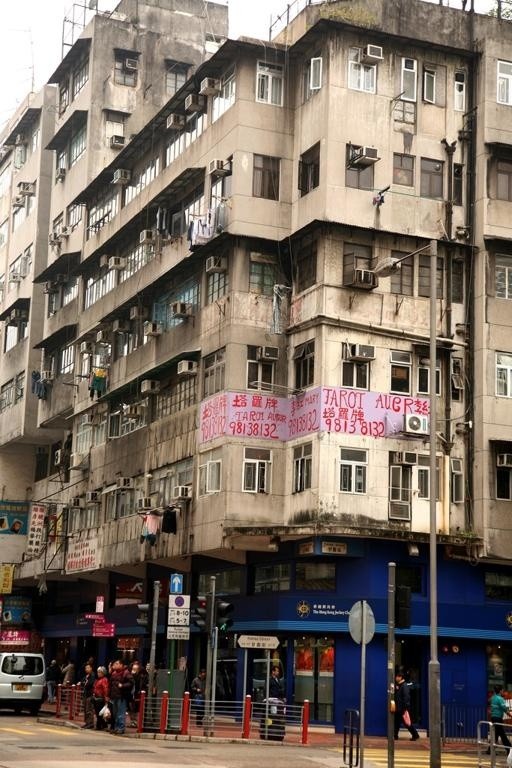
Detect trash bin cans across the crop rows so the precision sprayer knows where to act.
[260,698,286,741]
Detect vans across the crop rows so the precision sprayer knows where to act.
[0,651,48,715]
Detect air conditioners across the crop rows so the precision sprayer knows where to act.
[11,196,24,207]
[17,181,34,196]
[258,346,281,361]
[54,167,66,179]
[129,306,148,320]
[110,134,126,150]
[198,76,220,98]
[123,57,140,73]
[57,225,72,238]
[364,45,383,62]
[346,343,377,364]
[403,412,429,436]
[140,380,160,395]
[208,159,231,176]
[352,146,378,169]
[48,232,62,244]
[40,370,54,383]
[108,256,125,271]
[69,497,85,509]
[80,413,99,427]
[95,330,113,344]
[184,93,204,113]
[54,449,66,466]
[165,112,185,132]
[10,271,21,283]
[205,255,227,274]
[171,301,192,318]
[123,404,142,419]
[137,497,150,514]
[85,492,101,504]
[352,268,378,290]
[139,229,155,244]
[112,168,132,185]
[143,321,163,337]
[112,319,129,333]
[172,486,194,501]
[68,453,84,469]
[116,477,133,490]
[177,359,198,377]
[79,341,93,354]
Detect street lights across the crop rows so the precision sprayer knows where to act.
[369,230,445,767]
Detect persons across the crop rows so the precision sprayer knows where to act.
[10,521,22,534]
[45,650,207,735]
[394,671,420,741]
[483,683,512,756]
[22,613,29,621]
[0,518,8,530]
[269,665,281,700]
[506,749,512,766]
[5,613,10,619]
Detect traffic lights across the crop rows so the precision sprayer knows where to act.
[216,599,234,632]
[190,591,208,627]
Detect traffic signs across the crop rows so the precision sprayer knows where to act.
[237,633,281,649]
[84,613,105,619]
[92,622,115,627]
[92,631,115,636]
[94,619,105,622]
[92,627,115,631]
